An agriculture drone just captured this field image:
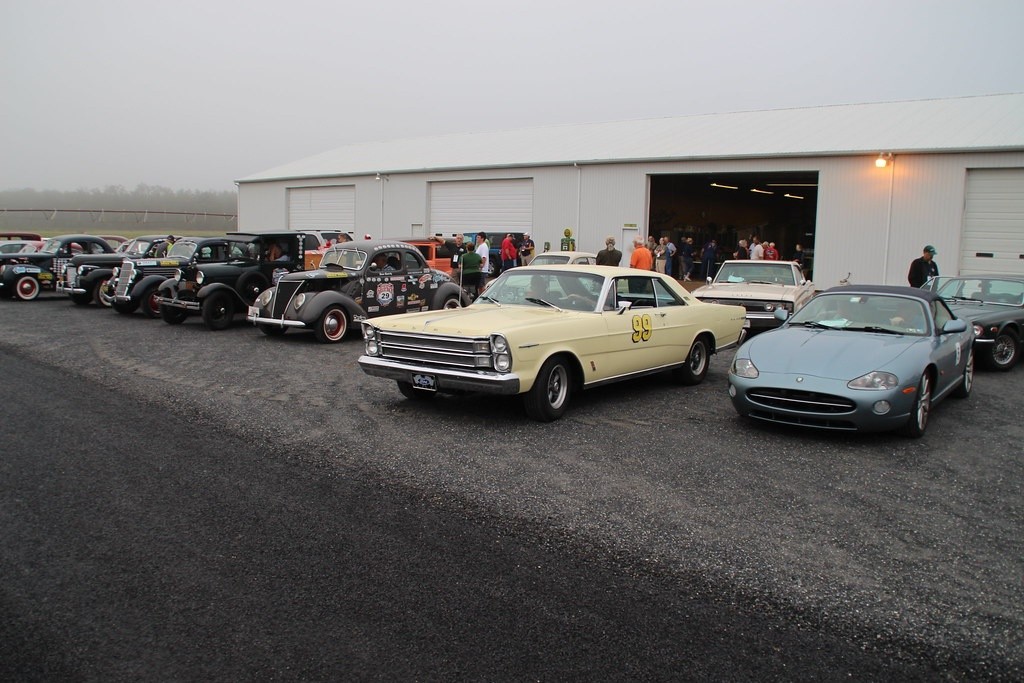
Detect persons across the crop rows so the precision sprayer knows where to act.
[154,235,174,258]
[338,234,350,242]
[370,253,396,272]
[889,302,919,330]
[646,234,804,283]
[501,232,535,271]
[427,231,489,296]
[596,237,622,266]
[524,275,614,310]
[630,235,653,270]
[268,244,288,262]
[908,245,939,289]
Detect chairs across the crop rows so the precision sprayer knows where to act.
[634,299,664,306]
[532,276,549,291]
[388,256,398,270]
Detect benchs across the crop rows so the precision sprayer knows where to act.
[973,292,1023,304]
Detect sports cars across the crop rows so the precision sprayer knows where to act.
[727,284,977,437]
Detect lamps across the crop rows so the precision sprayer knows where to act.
[375,172,380,180]
[876,152,892,167]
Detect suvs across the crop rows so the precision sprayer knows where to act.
[464,232,528,278]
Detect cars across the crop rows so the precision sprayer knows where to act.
[920,275,1024,371]
[692,260,815,331]
[484,251,627,296]
[107,236,247,319]
[0,232,130,302]
[246,240,472,344]
[358,265,746,423]
[56,235,173,308]
[153,231,305,329]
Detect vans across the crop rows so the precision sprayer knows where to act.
[303,230,355,251]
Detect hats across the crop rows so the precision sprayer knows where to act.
[681,237,686,240]
[167,235,174,241]
[364,234,371,240]
[687,238,693,241]
[523,232,530,238]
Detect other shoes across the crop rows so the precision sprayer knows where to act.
[679,275,691,281]
